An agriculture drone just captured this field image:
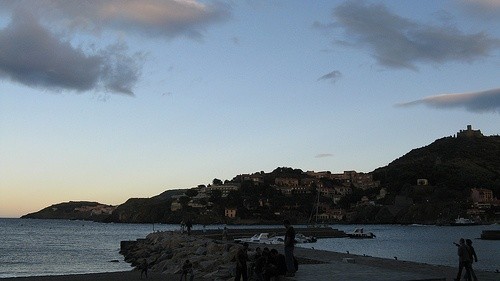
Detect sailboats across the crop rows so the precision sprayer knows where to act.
[306,181,332,229]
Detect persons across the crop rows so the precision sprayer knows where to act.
[249,246,285,281]
[282,221,299,272]
[453,238,478,281]
[235,243,249,281]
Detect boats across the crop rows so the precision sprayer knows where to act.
[450,215,476,225]
[346,227,376,238]
[232,231,318,245]
